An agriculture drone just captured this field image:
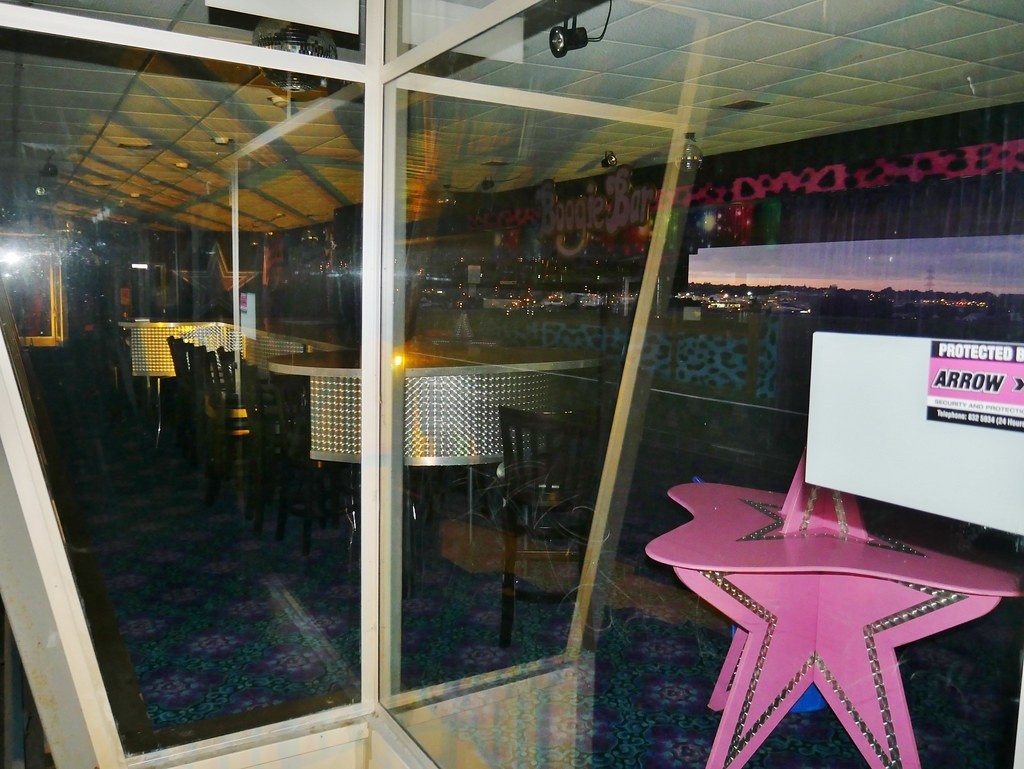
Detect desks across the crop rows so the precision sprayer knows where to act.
[265,347,611,542]
[119,318,239,445]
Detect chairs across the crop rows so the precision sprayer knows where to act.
[495,405,609,648]
[166,335,360,557]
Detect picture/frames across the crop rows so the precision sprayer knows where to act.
[0,225,68,348]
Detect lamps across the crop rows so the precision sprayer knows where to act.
[482,176,494,190]
[549,0,612,58]
[601,151,617,168]
